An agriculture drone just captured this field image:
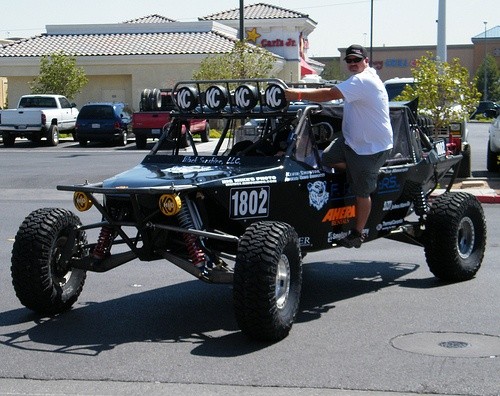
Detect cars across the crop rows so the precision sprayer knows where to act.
[72,102,132,144]
[474,97,500,120]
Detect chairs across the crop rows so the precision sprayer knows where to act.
[228,140,255,157]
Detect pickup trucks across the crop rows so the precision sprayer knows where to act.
[0,94,79,146]
[132,88,210,146]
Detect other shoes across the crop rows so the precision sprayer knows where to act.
[341,234,362,248]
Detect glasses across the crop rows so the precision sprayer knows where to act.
[346,58,362,63]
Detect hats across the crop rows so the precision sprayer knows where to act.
[343,45,367,60]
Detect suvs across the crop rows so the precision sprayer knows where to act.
[487,102,500,176]
[384,78,472,178]
[9,77,489,345]
